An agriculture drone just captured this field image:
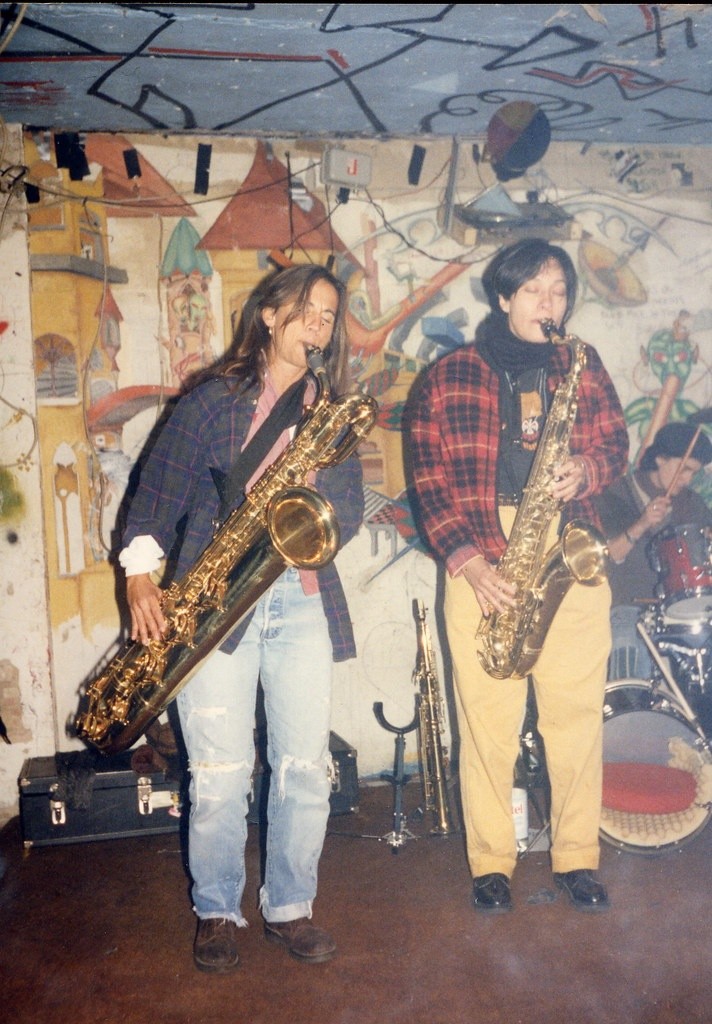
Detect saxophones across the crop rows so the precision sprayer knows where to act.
[471,312,616,683]
[410,597,457,838]
[70,340,383,759]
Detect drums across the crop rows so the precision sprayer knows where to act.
[598,678,712,858]
[646,523,712,620]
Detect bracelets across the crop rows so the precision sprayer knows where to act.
[625,529,638,545]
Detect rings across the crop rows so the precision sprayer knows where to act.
[653,504,656,510]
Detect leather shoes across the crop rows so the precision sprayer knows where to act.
[473,873,513,914]
[267,919,339,965]
[194,918,239,974]
[553,869,610,912]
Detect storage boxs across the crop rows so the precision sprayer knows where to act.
[18,731,361,847]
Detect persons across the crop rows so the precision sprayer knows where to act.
[598,423,712,687]
[118,264,365,973]
[401,237,630,914]
